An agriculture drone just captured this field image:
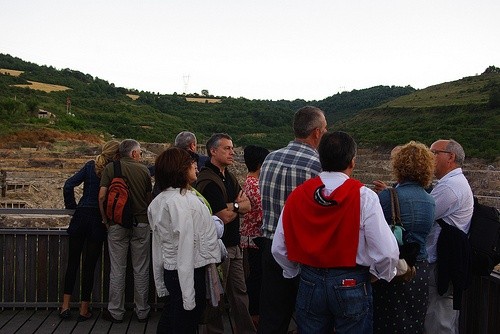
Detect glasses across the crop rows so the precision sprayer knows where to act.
[429,149,452,156]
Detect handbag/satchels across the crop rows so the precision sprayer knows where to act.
[106,159,139,230]
[467,196,500,277]
[384,186,409,249]
[435,218,478,277]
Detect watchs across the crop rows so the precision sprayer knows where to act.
[233,202,239,212]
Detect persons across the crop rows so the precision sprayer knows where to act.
[187,151,228,333]
[372,140,436,334]
[372,138,474,333]
[271,131,412,333]
[149,131,210,199]
[147,148,225,334]
[60,141,120,322]
[242,145,271,334]
[195,133,257,334]
[98,139,153,323]
[259,106,328,333]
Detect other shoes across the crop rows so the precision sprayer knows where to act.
[102,311,122,323]
[78,311,94,321]
[59,306,71,319]
[138,313,151,322]
